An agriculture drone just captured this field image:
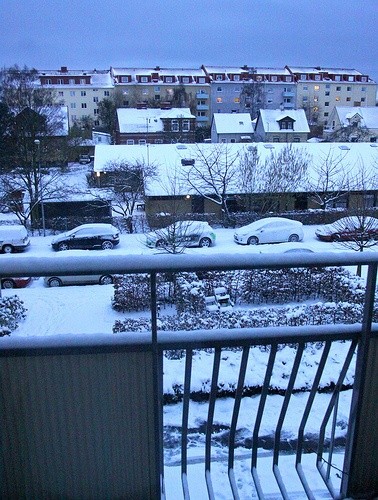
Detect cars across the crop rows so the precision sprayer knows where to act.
[44,273,116,288]
[234,217,304,245]
[146,221,216,249]
[0,276,32,289]
[315,216,378,242]
[79,154,90,164]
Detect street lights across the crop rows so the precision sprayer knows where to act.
[34,140,46,237]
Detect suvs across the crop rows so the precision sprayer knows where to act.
[0,226,31,253]
[51,223,120,251]
